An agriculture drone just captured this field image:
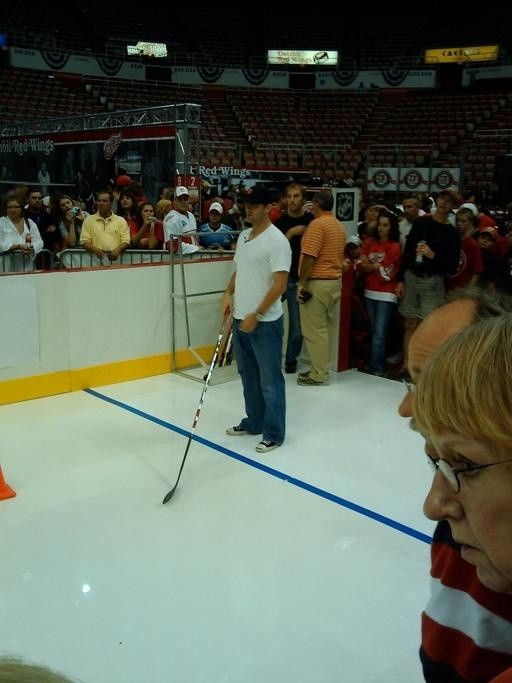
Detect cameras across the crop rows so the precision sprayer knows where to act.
[70,206,79,217]
[147,216,157,223]
[301,292,312,304]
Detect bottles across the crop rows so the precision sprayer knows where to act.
[415,242,424,262]
[26,232,31,248]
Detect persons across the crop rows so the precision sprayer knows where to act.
[2,161,250,272]
[270,183,344,386]
[217,182,294,454]
[346,186,512,382]
[395,285,512,683]
[410,313,510,597]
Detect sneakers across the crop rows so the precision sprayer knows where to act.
[255,440,279,453]
[375,352,411,382]
[226,426,251,434]
[285,362,329,386]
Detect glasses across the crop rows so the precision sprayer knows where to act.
[426,453,512,494]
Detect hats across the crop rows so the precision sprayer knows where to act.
[439,189,499,240]
[237,185,275,205]
[174,185,189,198]
[208,202,224,214]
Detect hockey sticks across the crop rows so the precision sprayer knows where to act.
[161,305,232,504]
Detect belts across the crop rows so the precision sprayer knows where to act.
[308,278,339,281]
